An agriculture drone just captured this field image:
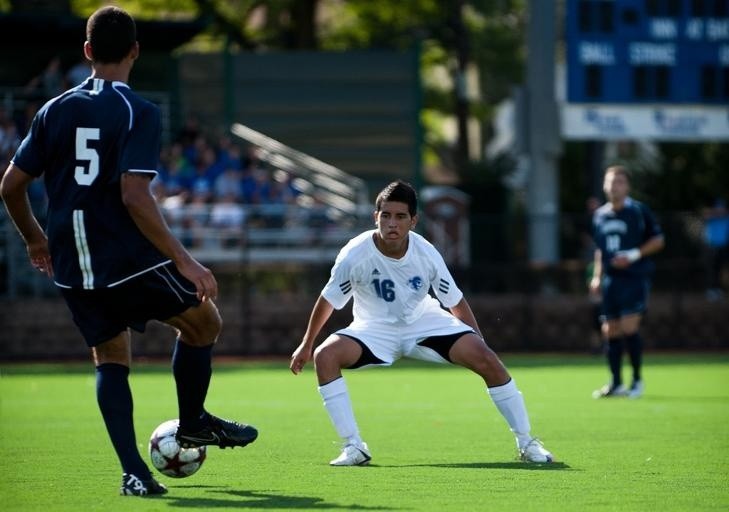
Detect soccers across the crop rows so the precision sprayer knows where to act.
[148,419,206,478]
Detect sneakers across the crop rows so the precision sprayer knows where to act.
[330,442,372,466]
[518,441,554,463]
[120,472,168,495]
[176,415,258,448]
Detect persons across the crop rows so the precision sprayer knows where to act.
[289,181,557,467]
[1,6,259,499]
[590,167,665,399]
